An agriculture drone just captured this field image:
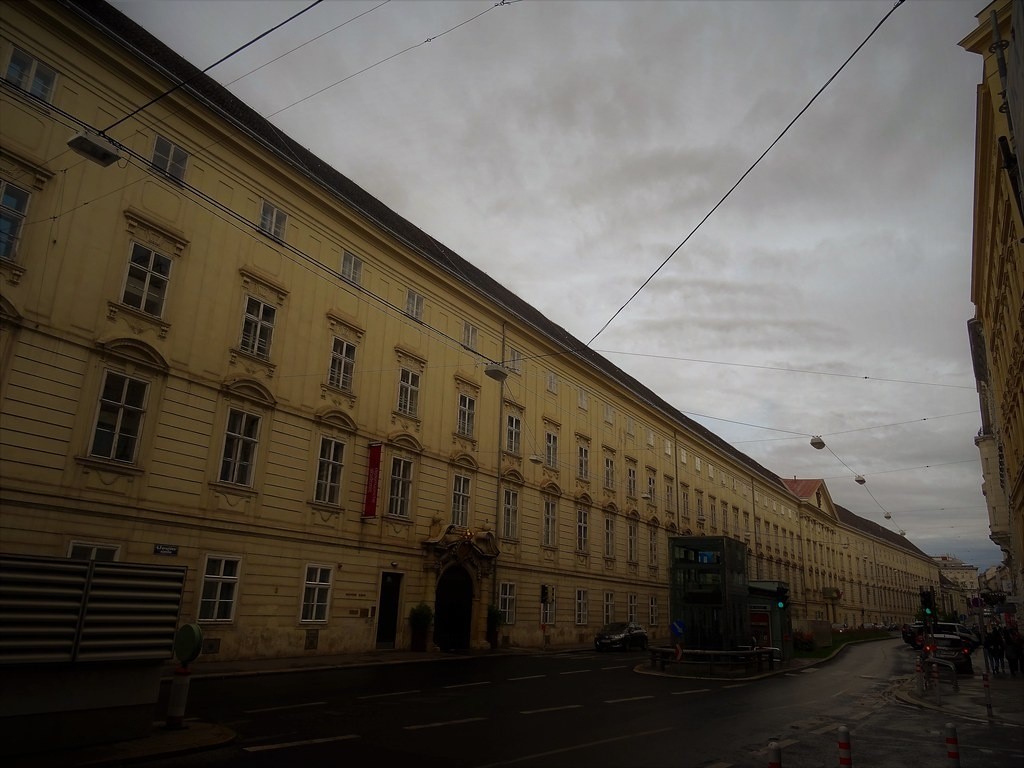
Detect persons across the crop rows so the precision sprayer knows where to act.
[984,626,1024,676]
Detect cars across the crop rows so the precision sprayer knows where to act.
[904,619,979,649]
[833,621,902,635]
[921,634,973,674]
[594,621,650,651]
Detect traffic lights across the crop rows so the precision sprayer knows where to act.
[920,590,934,618]
[778,587,791,609]
